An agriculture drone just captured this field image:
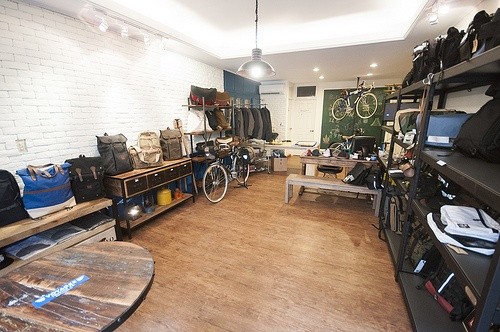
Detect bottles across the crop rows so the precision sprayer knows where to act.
[157,187,172,205]
[174,189,181,199]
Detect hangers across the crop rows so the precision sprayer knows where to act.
[235,104,266,109]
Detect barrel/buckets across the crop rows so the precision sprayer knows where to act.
[142,193,154,213]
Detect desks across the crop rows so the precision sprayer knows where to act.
[0,241,155,332]
[298,151,380,209]
[252,141,320,175]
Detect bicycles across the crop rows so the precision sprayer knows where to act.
[201,144,251,202]
[331,81,378,120]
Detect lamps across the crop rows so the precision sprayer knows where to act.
[235,0,276,77]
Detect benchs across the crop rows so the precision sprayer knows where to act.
[284,173,382,218]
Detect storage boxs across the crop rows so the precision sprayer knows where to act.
[271,154,291,172]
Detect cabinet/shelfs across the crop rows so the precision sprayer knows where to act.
[378,45,500,332]
[104,157,195,240]
[181,96,236,192]
[0,198,117,277]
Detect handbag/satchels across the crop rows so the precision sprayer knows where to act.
[216,91,230,106]
[128,146,164,168]
[394,149,462,203]
[190,86,216,110]
[416,257,475,322]
[402,9,500,88]
[344,163,381,190]
[65,155,106,202]
[0,169,29,226]
[12,163,76,219]
[384,102,477,148]
[437,93,499,163]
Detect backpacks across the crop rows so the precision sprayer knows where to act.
[384,185,441,278]
[160,127,183,160]
[138,130,160,148]
[96,132,133,176]
[427,205,500,256]
